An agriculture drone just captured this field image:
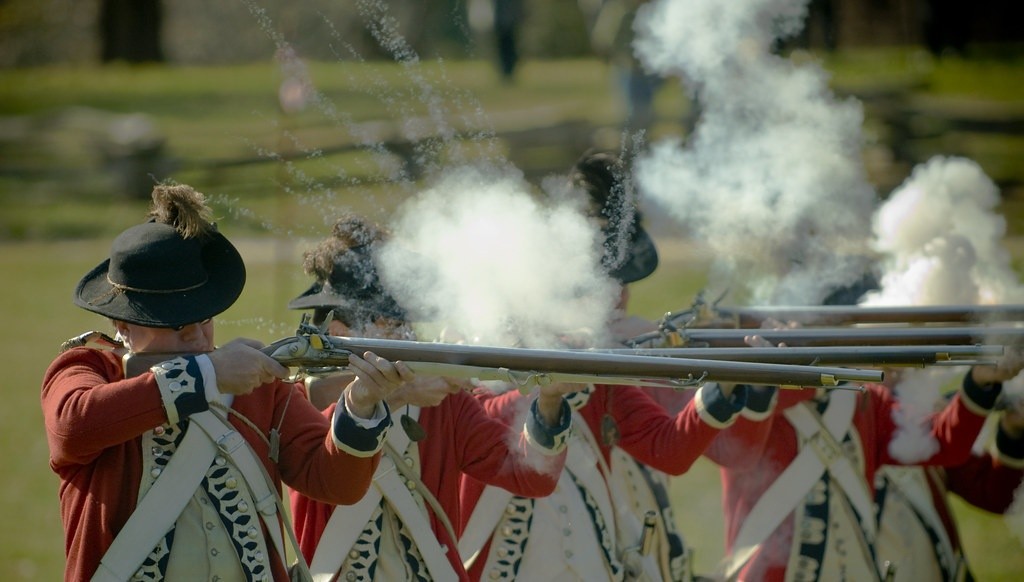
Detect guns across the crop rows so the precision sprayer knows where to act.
[303,285,1024,415]
[121,310,888,394]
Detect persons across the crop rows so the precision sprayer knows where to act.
[39,181,414,581]
[459,119,1024,582]
[290,215,586,582]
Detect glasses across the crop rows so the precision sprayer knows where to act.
[334,307,404,330]
[172,318,211,330]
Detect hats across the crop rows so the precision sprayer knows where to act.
[288,213,442,323]
[545,151,659,284]
[72,185,246,327]
